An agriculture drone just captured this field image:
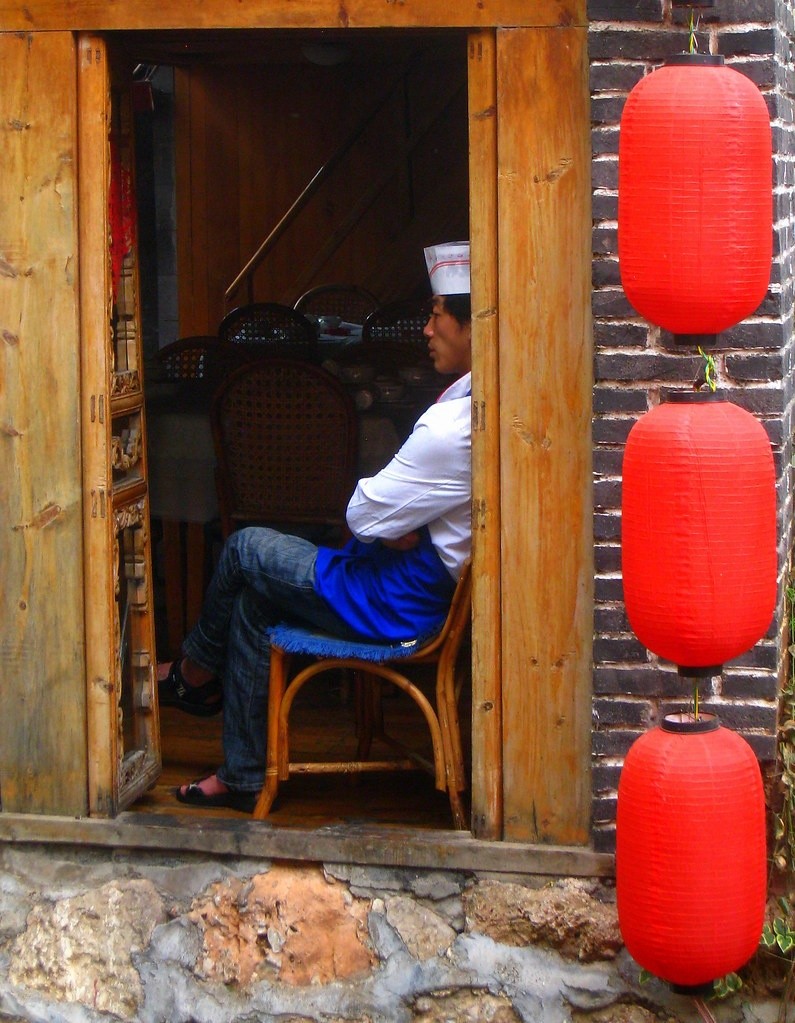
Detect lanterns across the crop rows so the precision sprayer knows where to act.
[622,389,778,675]
[616,52,772,349]
[616,714,767,998]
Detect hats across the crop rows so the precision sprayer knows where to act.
[423,240,472,295]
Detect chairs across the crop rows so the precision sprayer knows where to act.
[145,277,453,785]
[255,557,477,829]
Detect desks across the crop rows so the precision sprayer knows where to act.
[229,316,430,352]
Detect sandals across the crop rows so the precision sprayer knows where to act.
[156,653,226,716]
[174,771,281,812]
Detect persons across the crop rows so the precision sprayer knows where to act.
[152,237,471,806]
